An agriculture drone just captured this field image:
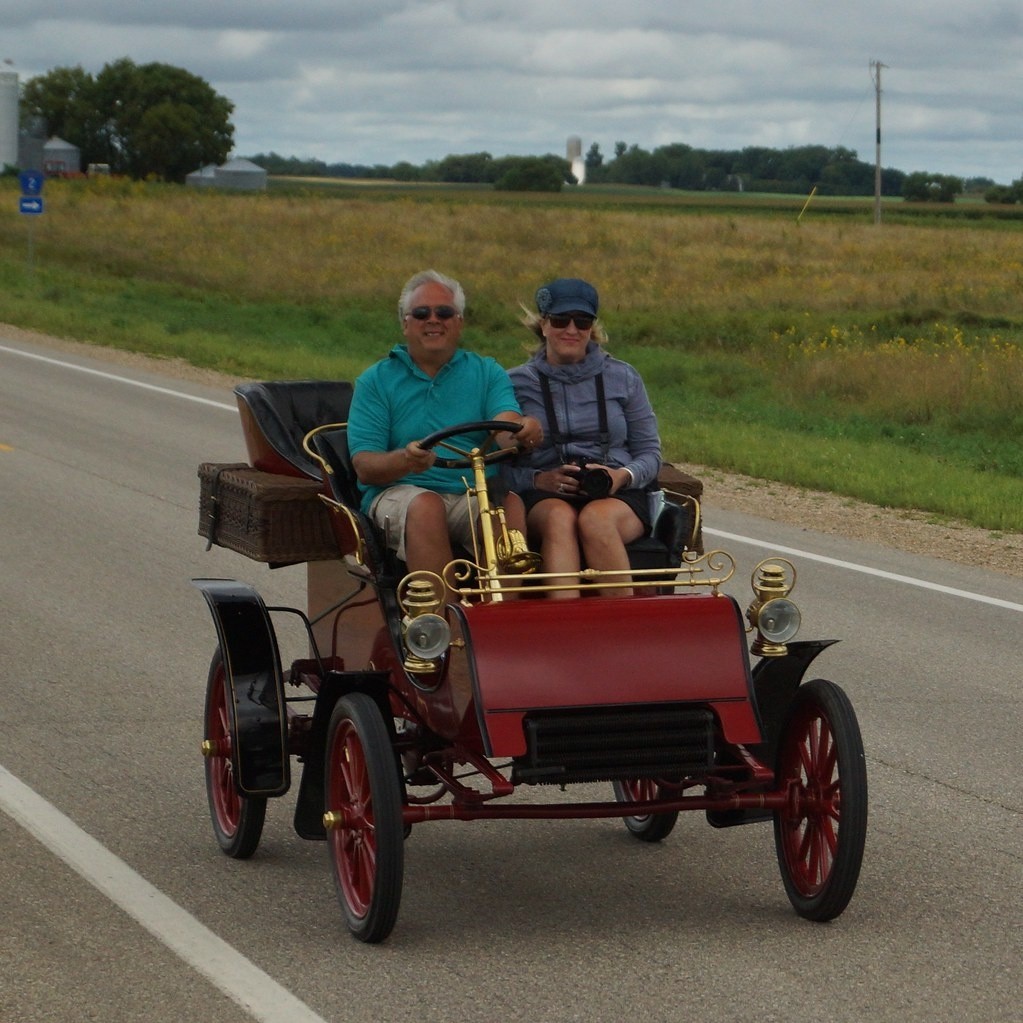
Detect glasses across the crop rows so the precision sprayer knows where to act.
[545,312,594,331]
[406,305,461,320]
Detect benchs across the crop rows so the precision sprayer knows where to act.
[230,380,355,482]
[302,422,700,588]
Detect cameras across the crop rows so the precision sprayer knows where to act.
[565,456,613,498]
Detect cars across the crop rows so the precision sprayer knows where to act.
[191,380,870,943]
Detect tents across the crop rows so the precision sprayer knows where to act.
[186,157,267,190]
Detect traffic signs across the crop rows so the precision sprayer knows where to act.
[19,168,44,213]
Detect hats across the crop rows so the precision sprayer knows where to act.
[535,278,600,321]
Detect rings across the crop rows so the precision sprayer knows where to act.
[530,440,535,445]
[558,483,565,493]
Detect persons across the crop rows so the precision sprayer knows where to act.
[505,279,662,598]
[347,269,543,623]
